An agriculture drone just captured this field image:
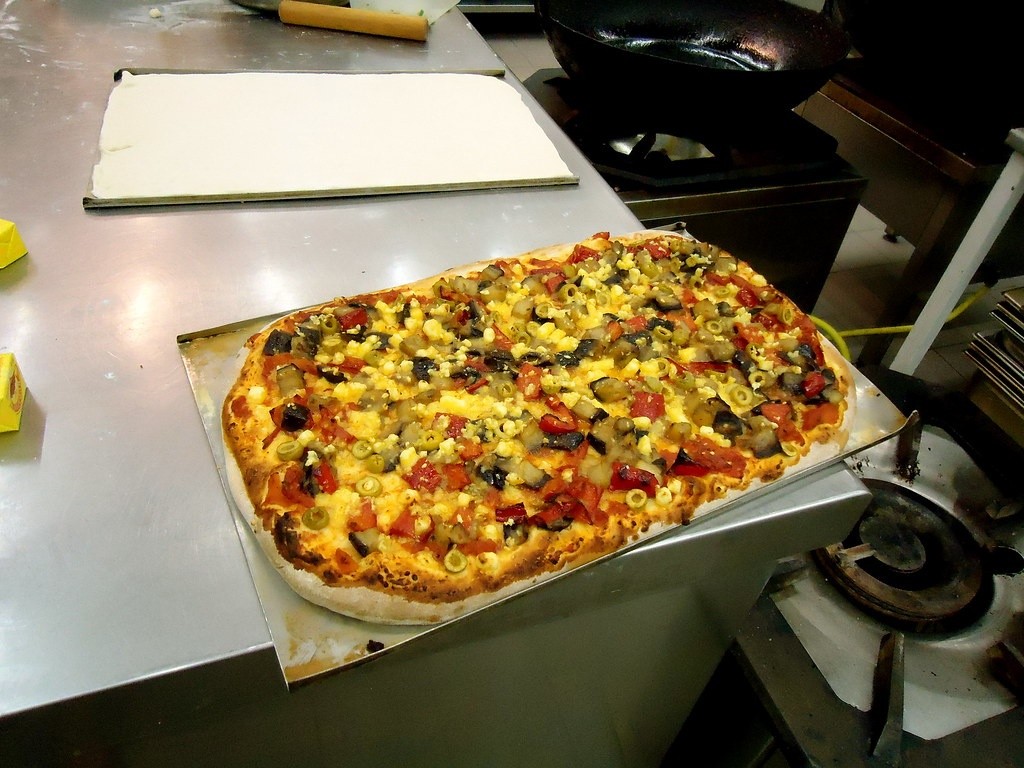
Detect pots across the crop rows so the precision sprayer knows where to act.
[539,0,853,123]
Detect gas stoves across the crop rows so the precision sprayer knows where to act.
[740,425,1024,768]
[526,65,869,315]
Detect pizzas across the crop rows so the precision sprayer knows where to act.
[213,228,856,624]
